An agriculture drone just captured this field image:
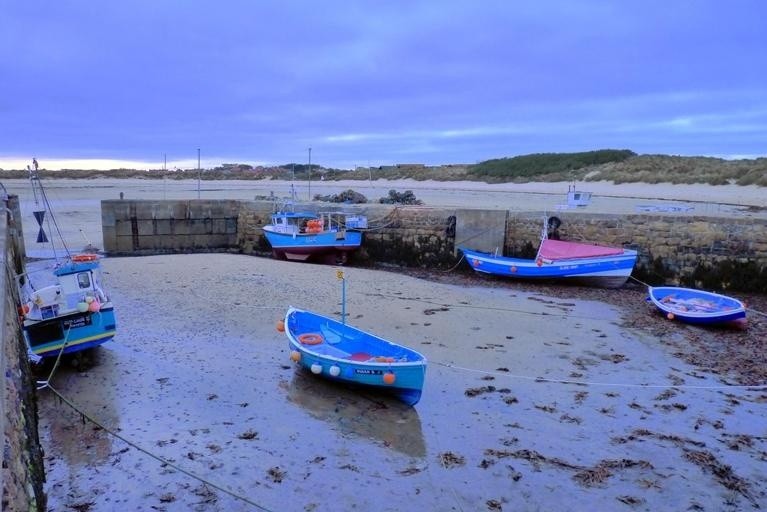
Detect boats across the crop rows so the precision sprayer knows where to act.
[13,157,118,372]
[556,182,593,208]
[277,277,427,411]
[260,182,370,264]
[456,228,639,291]
[646,282,747,330]
